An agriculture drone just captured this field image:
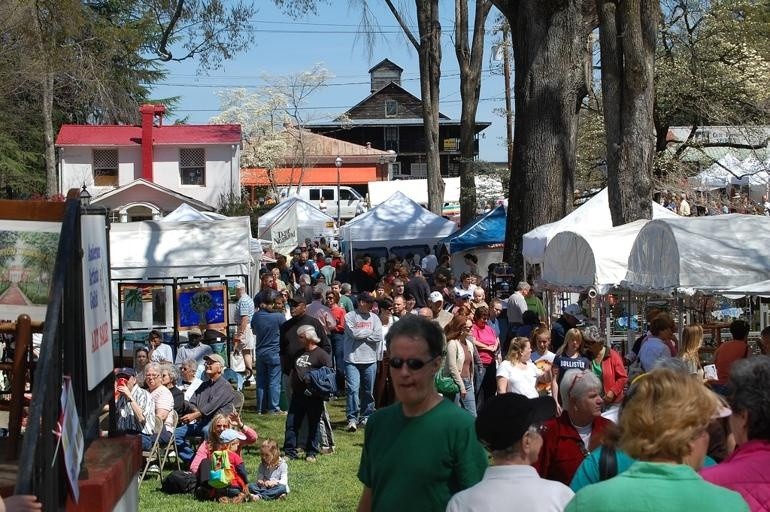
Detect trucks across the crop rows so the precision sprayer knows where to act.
[278,185,365,220]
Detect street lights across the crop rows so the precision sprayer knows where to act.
[379,155,386,182]
[335,154,343,229]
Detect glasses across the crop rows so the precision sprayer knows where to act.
[145,373,159,378]
[180,367,187,371]
[568,371,585,395]
[204,360,214,366]
[387,308,393,311]
[326,296,333,300]
[387,356,436,370]
[536,424,549,433]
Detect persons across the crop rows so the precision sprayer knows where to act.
[355,315,488,512]
[445,392,575,512]
[443,315,478,419]
[714,319,753,379]
[308,251,320,282]
[470,287,489,313]
[271,267,286,291]
[427,290,454,328]
[622,306,663,365]
[495,336,559,400]
[392,294,407,320]
[176,357,204,400]
[290,249,302,269]
[352,237,413,284]
[550,326,589,417]
[341,282,358,308]
[582,324,627,411]
[675,324,704,378]
[524,282,546,322]
[314,241,325,257]
[568,381,718,495]
[470,306,499,365]
[431,271,453,296]
[330,280,354,313]
[487,298,503,368]
[282,324,333,462]
[384,278,405,308]
[316,253,325,270]
[174,328,214,380]
[296,273,311,296]
[144,362,174,471]
[253,274,281,311]
[279,191,368,217]
[291,252,316,295]
[532,367,617,486]
[454,271,477,299]
[530,324,557,371]
[451,289,471,315]
[375,282,386,303]
[320,257,336,286]
[275,254,294,285]
[209,428,250,503]
[657,189,770,217]
[326,289,346,396]
[404,292,419,315]
[190,411,258,500]
[304,283,337,400]
[279,295,336,456]
[421,246,438,277]
[434,254,454,286]
[507,281,531,329]
[233,282,255,385]
[698,355,770,512]
[144,329,174,366]
[248,437,291,502]
[636,310,674,371]
[250,293,287,415]
[280,289,293,320]
[331,253,343,267]
[374,298,399,408]
[342,292,383,431]
[311,273,326,287]
[563,367,751,512]
[550,304,587,353]
[319,237,328,254]
[274,294,286,313]
[463,253,480,274]
[133,347,149,389]
[174,353,236,466]
[115,366,156,451]
[517,310,540,337]
[406,263,431,309]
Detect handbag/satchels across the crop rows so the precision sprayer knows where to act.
[229,345,247,373]
[434,340,460,394]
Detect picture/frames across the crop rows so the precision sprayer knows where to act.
[124,288,143,322]
[228,279,241,302]
[175,285,228,331]
[151,287,166,326]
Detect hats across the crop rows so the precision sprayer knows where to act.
[218,428,247,444]
[454,289,471,299]
[328,280,341,287]
[428,291,443,303]
[187,327,201,336]
[474,392,557,452]
[287,296,307,304]
[116,367,136,377]
[234,282,245,288]
[357,291,377,302]
[203,353,224,367]
[563,303,587,322]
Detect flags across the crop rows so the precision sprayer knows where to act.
[269,200,299,255]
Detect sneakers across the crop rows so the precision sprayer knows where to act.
[347,422,357,432]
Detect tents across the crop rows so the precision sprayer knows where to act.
[704,151,770,203]
[256,196,339,256]
[440,204,508,278]
[107,215,254,379]
[337,190,459,262]
[542,217,652,348]
[161,201,274,295]
[622,211,770,350]
[520,186,683,289]
[687,149,742,191]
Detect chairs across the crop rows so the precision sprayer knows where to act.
[138,415,163,489]
[186,391,244,466]
[158,409,181,480]
